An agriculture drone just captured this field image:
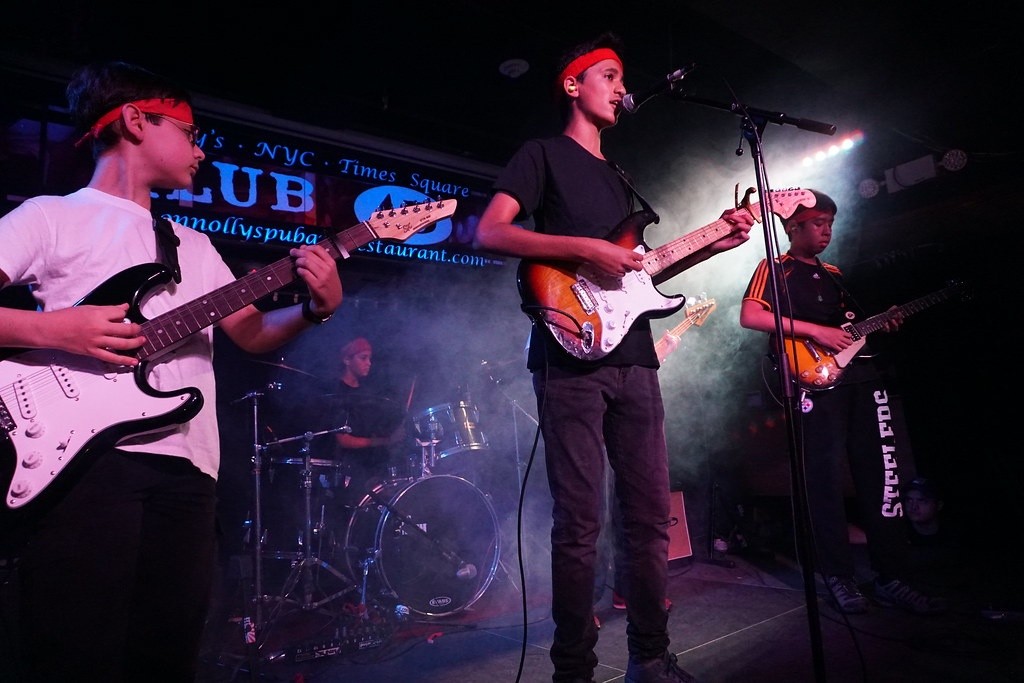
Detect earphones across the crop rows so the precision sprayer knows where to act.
[568,86,576,91]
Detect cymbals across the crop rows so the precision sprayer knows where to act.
[301,392,401,419]
[247,358,321,382]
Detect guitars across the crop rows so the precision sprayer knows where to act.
[515,185,819,370]
[0,192,460,522]
[767,277,972,395]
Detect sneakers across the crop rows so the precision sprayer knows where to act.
[875,571,951,619]
[821,576,872,615]
[623,651,698,683]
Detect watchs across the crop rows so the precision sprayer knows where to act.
[301,295,336,327]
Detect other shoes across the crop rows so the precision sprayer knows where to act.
[591,614,602,630]
[339,592,397,625]
[610,589,672,612]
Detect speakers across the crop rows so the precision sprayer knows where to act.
[665,487,696,562]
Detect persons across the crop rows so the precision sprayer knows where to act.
[472,30,753,683]
[739,190,913,621]
[588,329,681,632]
[0,41,344,683]
[329,328,413,468]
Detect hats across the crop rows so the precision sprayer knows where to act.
[901,474,934,497]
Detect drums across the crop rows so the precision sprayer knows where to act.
[344,474,501,620]
[406,399,493,475]
[259,454,343,561]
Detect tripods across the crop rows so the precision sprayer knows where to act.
[357,486,477,660]
[255,413,390,652]
[219,381,304,646]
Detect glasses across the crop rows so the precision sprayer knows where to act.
[903,494,930,505]
[142,110,201,145]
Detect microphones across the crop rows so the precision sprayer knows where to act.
[457,565,478,580]
[619,63,697,115]
